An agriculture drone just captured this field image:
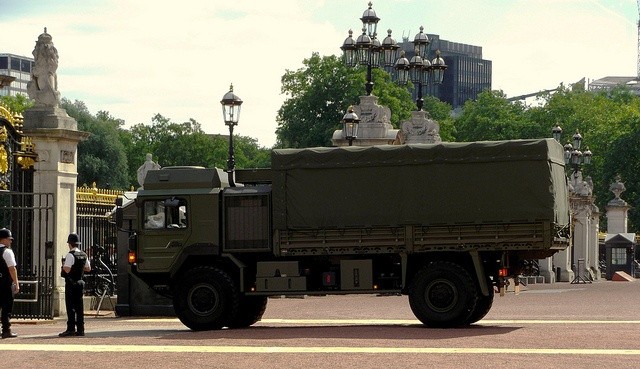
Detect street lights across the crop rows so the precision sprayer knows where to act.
[220,83,243,187]
[340,2,400,96]
[342,106,360,146]
[394,26,449,111]
[552,122,592,198]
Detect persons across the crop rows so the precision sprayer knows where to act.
[0,228,20,338]
[60,235,91,337]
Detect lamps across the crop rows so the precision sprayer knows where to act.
[127,251,136,264]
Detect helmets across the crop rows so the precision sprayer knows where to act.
[67,234,82,244]
[0,228,13,238]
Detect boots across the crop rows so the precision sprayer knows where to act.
[1,325,17,338]
[59,322,76,337]
[75,321,85,335]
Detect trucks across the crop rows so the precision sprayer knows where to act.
[115,138,571,331]
